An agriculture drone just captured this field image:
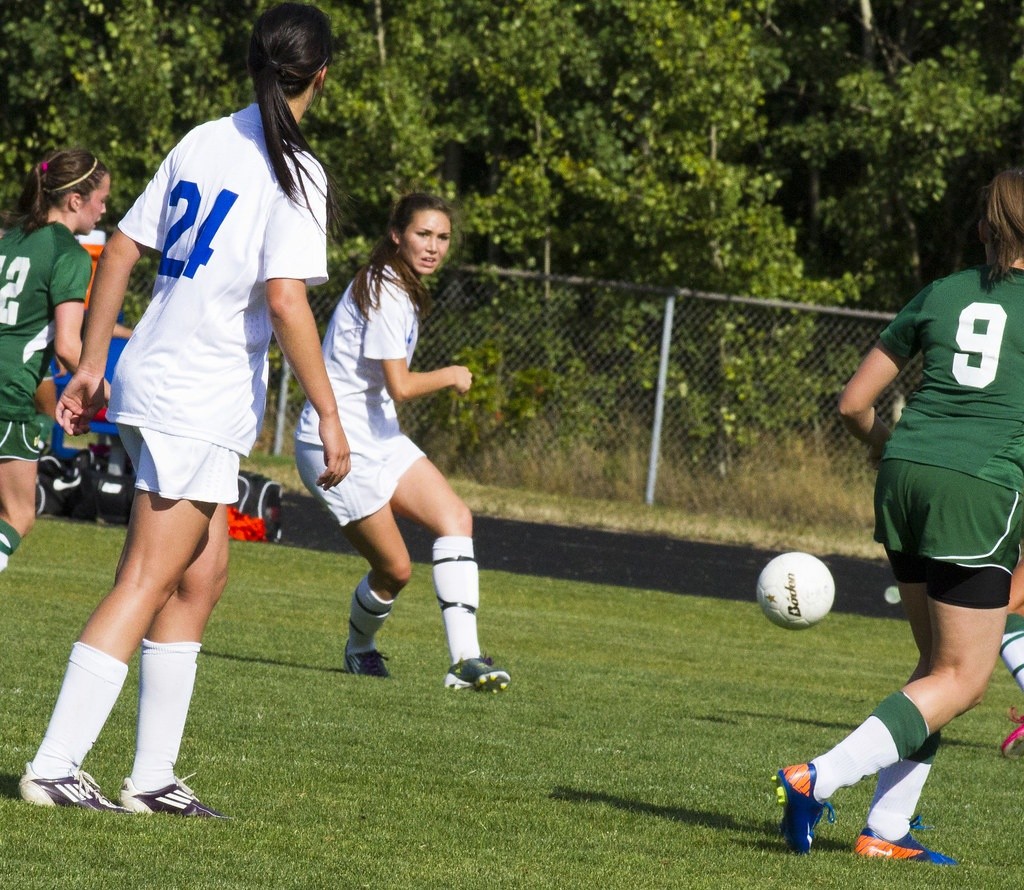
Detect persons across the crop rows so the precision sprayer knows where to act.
[0,151,111,573]
[770,170,1024,863]
[1001,538,1024,759]
[293,192,509,695]
[52,301,134,379]
[17,1,351,819]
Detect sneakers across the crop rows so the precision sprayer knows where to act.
[443,658,511,695]
[120,777,234,820]
[1001,716,1024,759]
[343,638,390,678]
[854,824,959,865]
[770,760,826,855]
[17,761,136,814]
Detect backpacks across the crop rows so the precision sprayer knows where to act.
[36,444,98,522]
[227,470,283,544]
[93,448,138,526]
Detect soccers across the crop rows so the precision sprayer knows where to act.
[756,552,835,631]
